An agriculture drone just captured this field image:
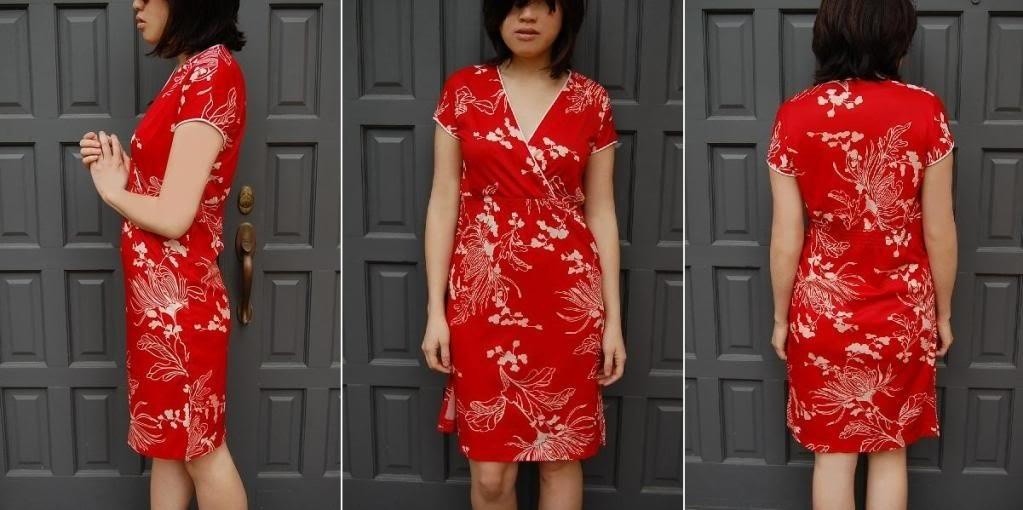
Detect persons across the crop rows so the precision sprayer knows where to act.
[421,0,628,510]
[80,1,249,510]
[765,0,959,510]
